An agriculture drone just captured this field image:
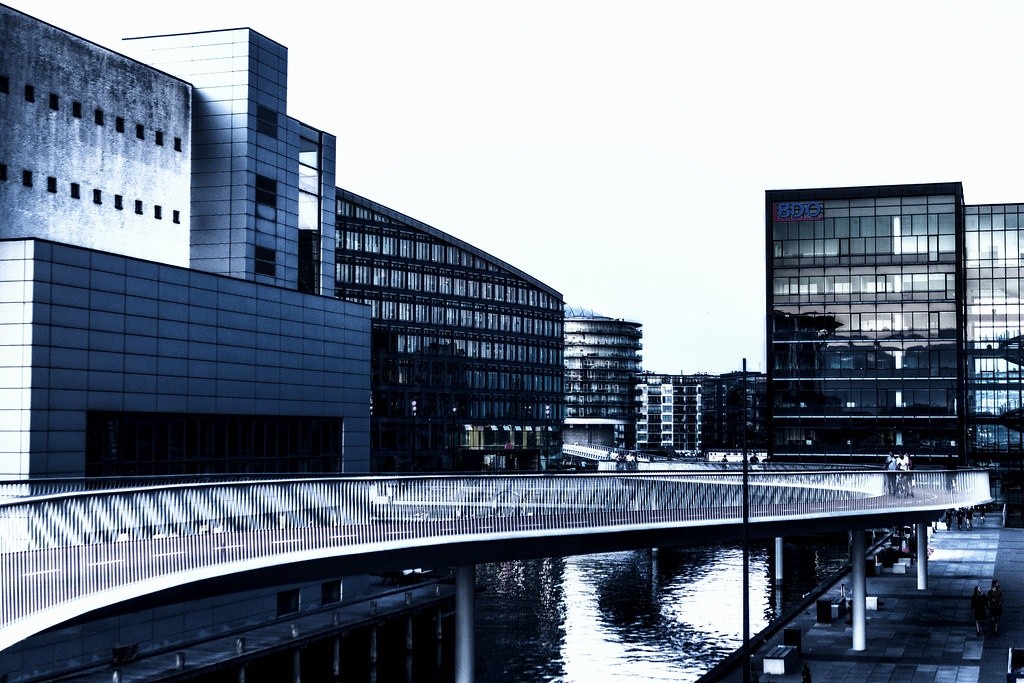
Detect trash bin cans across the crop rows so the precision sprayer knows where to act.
[816,597,833,624]
[784,627,802,659]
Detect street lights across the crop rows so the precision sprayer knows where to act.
[408,389,417,476]
[451,403,457,471]
[725,358,774,683]
[545,401,550,473]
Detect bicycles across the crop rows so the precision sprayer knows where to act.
[949,479,956,504]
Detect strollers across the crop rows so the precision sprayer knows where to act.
[895,465,914,498]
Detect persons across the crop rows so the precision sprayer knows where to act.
[721,455,728,470]
[890,527,913,565]
[800,659,812,683]
[896,451,910,498]
[965,508,973,531]
[986,579,1003,633]
[581,459,587,471]
[616,451,639,471]
[762,456,772,462]
[750,452,759,463]
[977,504,987,523]
[969,586,990,638]
[883,451,897,498]
[904,452,914,497]
[946,509,953,531]
[956,508,965,531]
